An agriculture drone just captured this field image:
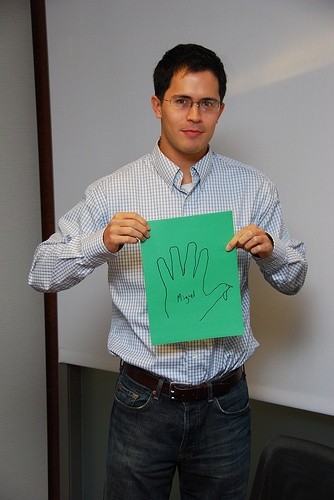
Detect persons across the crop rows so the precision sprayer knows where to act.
[27,44,309,500]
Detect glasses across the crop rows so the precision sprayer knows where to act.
[156,95,222,113]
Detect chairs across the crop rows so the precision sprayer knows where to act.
[250,435,334,500]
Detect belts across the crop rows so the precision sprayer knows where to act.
[122,361,243,402]
